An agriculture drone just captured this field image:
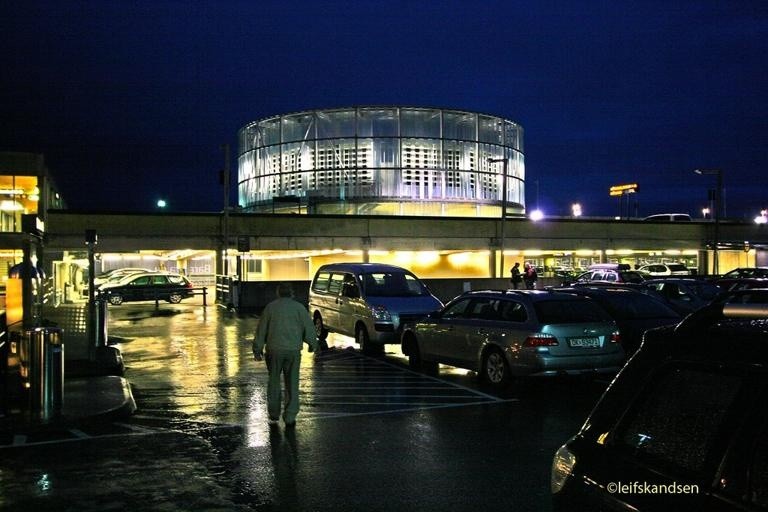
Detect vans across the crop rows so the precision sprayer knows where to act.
[639,213,693,222]
[303,257,447,359]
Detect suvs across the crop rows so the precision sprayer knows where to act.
[545,276,767,512]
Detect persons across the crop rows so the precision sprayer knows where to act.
[253,283,320,428]
[524,261,537,289]
[511,262,521,290]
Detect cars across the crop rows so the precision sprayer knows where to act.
[524,260,767,318]
[399,282,627,397]
[78,266,159,300]
[92,270,196,305]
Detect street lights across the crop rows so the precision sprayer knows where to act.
[81,226,99,366]
[690,166,724,275]
[217,141,233,214]
[484,156,508,278]
